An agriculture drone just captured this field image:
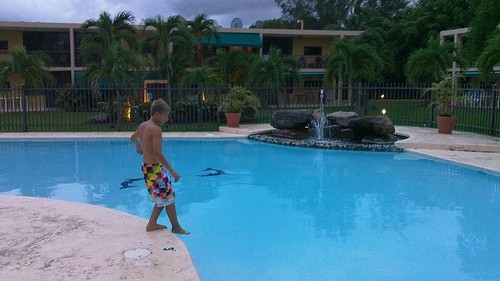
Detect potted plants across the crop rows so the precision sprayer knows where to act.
[220,86,259,127]
[420,75,470,133]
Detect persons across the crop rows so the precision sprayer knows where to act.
[130,98,191,234]
